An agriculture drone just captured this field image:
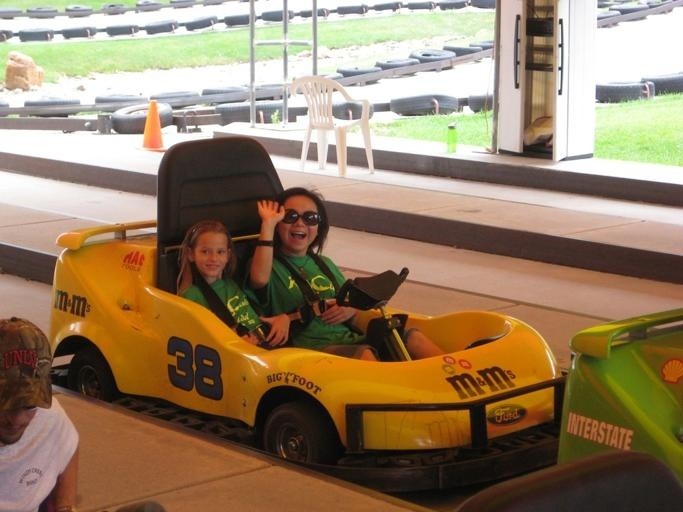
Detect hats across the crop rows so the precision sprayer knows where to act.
[0,317,52,413]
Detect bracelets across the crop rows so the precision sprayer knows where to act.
[255,238,275,247]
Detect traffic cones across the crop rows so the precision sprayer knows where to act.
[143,100,163,150]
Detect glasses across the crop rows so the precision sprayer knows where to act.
[281,208,322,226]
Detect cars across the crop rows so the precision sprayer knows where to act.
[556,297,682,480]
[53,136,562,468]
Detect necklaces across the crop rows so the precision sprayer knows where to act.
[283,253,311,271]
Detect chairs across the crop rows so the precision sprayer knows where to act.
[454,449,683,510]
[286,75,379,178]
[154,136,286,297]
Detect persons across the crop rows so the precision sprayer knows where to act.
[1,317,163,511]
[248,187,449,362]
[177,218,278,352]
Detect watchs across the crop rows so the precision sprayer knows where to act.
[56,504,77,512]
